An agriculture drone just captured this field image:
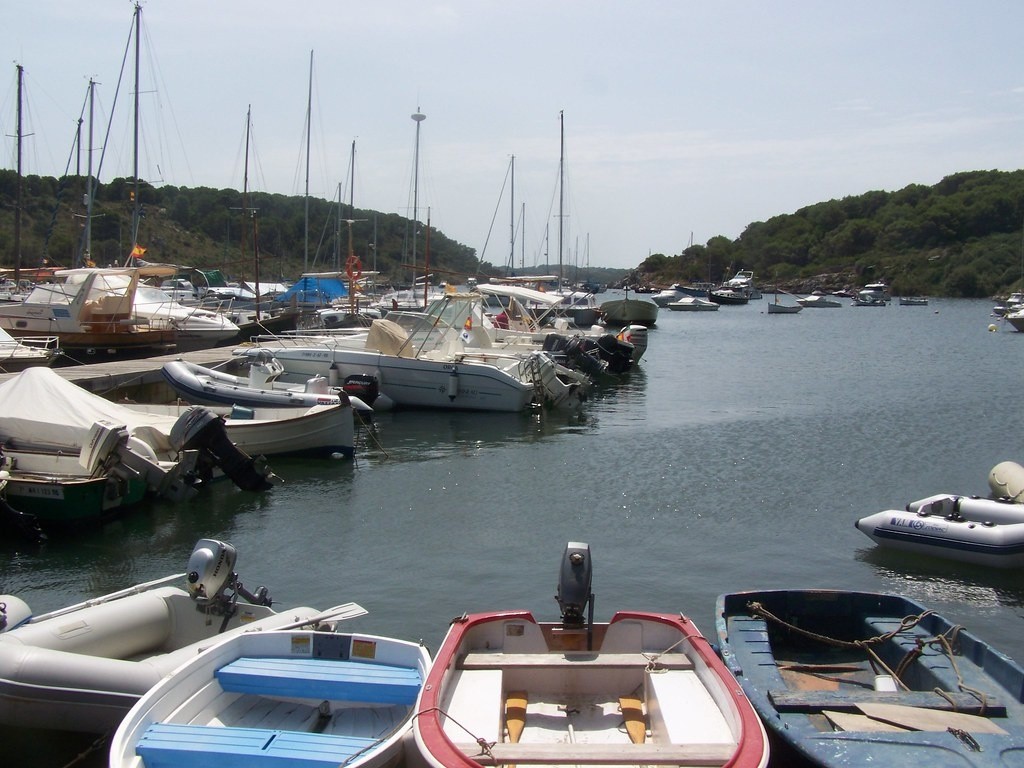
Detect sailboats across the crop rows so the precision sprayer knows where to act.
[1,0,708,523]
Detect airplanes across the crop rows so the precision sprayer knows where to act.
[859,281,892,302]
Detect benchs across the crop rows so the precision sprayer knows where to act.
[772,689,1007,711]
[462,652,692,674]
[215,656,421,709]
[137,725,379,766]
[452,743,739,764]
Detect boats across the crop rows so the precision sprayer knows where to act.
[767,270,803,314]
[108,627,433,768]
[709,261,762,305]
[796,295,842,307]
[854,493,1024,570]
[900,296,928,306]
[832,288,856,298]
[667,296,720,312]
[992,292,1024,333]
[0,537,276,738]
[811,291,827,296]
[850,294,885,307]
[715,588,1024,767]
[414,541,771,768]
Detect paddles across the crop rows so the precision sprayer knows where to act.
[197,601,370,654]
[617,696,648,768]
[505,688,531,768]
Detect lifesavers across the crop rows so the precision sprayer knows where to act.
[345,256,362,281]
